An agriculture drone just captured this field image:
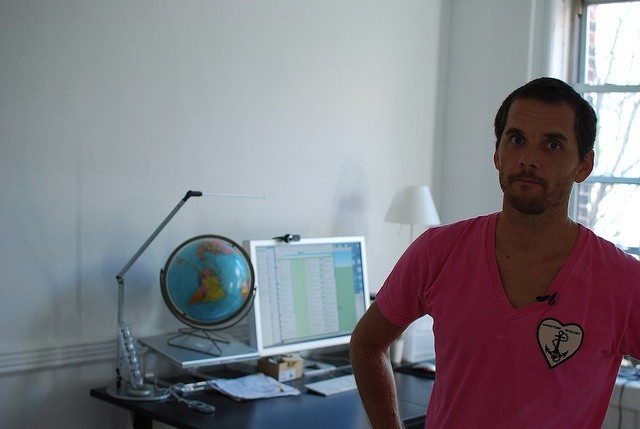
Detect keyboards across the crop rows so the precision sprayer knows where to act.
[303,372,359,400]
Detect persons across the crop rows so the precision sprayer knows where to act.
[349,77,640,428]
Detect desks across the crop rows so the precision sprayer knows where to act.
[87,353,436,429]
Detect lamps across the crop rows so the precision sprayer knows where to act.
[383,184,442,370]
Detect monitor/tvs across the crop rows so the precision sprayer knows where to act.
[239,234,372,361]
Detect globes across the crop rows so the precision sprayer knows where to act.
[159,234,254,356]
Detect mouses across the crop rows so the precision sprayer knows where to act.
[406,358,441,379]
